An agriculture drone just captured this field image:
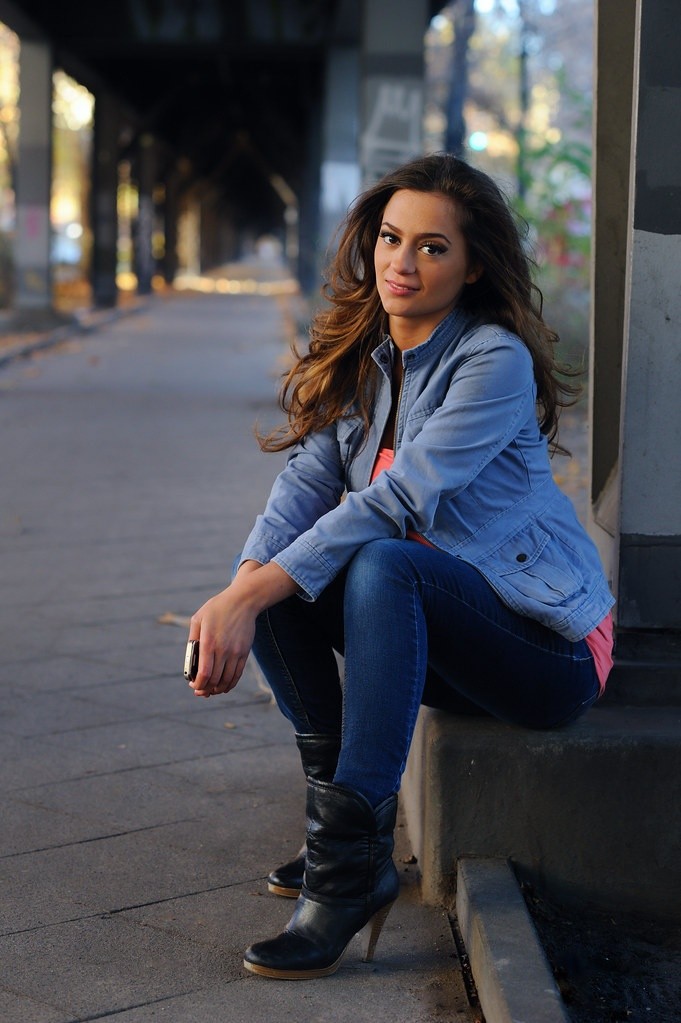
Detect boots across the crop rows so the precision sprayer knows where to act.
[243,778,403,980]
[266,725,350,899]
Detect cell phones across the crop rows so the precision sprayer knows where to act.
[183,639,200,683]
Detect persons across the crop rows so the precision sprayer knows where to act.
[186,155,616,981]
